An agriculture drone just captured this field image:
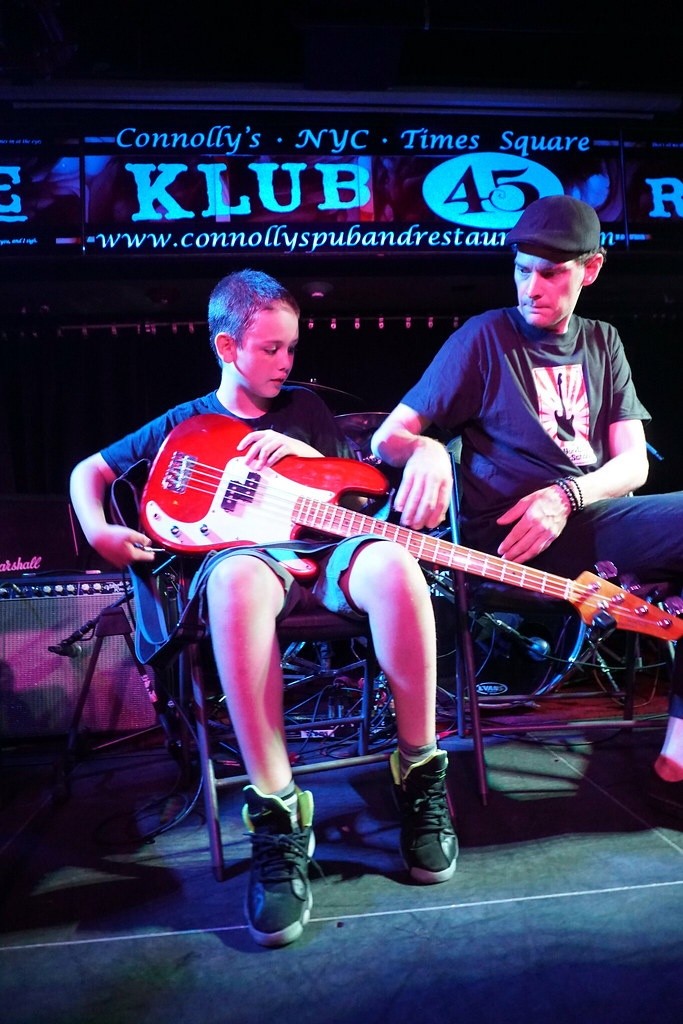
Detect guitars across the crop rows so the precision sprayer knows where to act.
[139,412,683,647]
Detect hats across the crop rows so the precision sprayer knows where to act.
[505,195,603,252]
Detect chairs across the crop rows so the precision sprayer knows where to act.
[99,411,683,881]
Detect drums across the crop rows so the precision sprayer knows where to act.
[416,559,588,710]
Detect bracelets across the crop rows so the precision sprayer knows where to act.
[564,477,584,511]
[548,478,579,513]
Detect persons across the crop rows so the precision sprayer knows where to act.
[371,196,683,803]
[70,269,458,948]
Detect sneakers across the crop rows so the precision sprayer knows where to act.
[386,748,460,883]
[242,786,316,947]
